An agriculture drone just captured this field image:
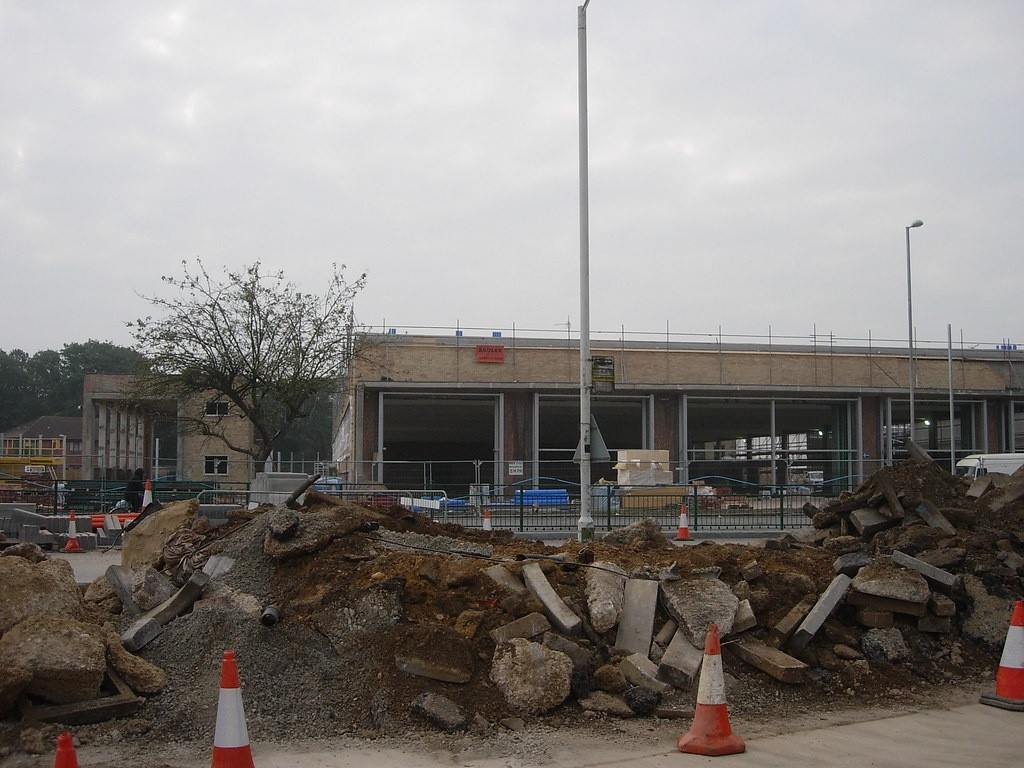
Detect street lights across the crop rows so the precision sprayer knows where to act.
[906,220,924,443]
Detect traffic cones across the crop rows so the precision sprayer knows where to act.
[979,600,1024,712]
[211,651,255,768]
[673,503,695,541]
[54,733,78,768]
[141,479,153,514]
[483,509,492,531]
[59,510,87,553]
[678,624,746,757]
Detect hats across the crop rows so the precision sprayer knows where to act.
[134,468,146,476]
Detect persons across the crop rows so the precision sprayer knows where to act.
[124,468,144,512]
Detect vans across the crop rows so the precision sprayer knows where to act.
[955,453,1024,479]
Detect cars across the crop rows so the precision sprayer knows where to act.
[804,471,823,490]
[313,477,358,501]
